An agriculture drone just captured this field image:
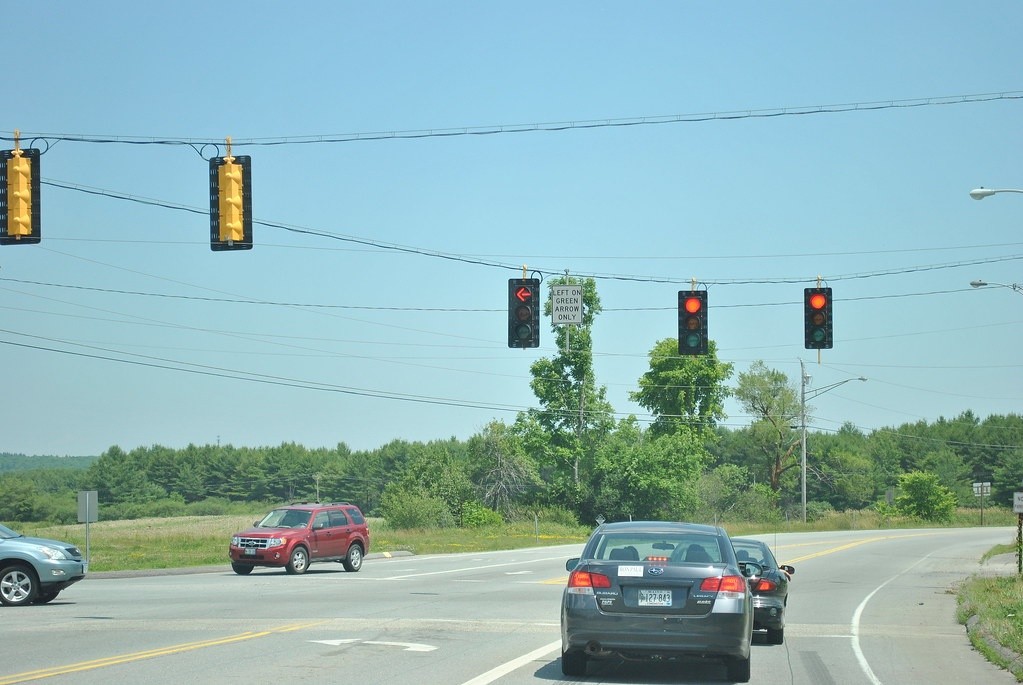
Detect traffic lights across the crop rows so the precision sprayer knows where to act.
[677,291,707,356]
[508,278,540,348]
[803,287,833,349]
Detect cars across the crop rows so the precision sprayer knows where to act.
[671,538,797,645]
[1,522,87,607]
[559,521,763,683]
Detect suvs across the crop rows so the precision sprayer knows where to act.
[229,500,370,577]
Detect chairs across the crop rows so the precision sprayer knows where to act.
[610,548,635,560]
[737,550,749,560]
[686,550,713,562]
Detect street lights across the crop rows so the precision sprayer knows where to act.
[800,357,868,524]
